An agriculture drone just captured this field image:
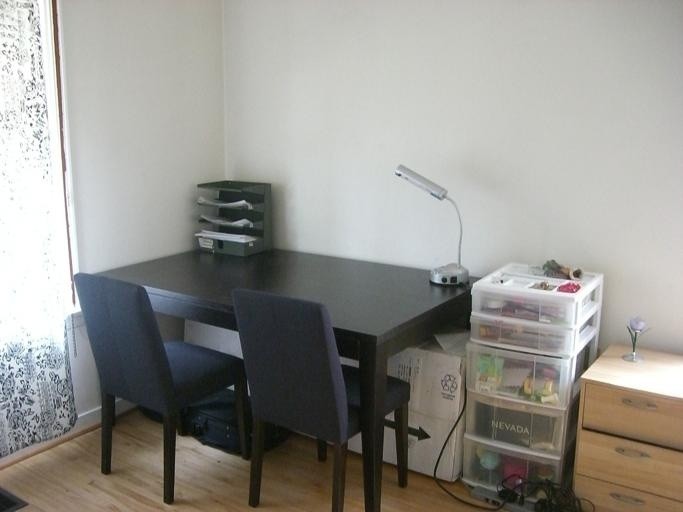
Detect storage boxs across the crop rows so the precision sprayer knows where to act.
[460,261,604,503]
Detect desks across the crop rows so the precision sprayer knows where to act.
[90,246,481,512]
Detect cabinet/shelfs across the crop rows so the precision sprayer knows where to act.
[570,341,683,511]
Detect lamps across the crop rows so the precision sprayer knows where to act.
[393,163,469,287]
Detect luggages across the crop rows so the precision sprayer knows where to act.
[192,390,289,457]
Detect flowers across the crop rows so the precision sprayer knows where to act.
[624,314,650,354]
